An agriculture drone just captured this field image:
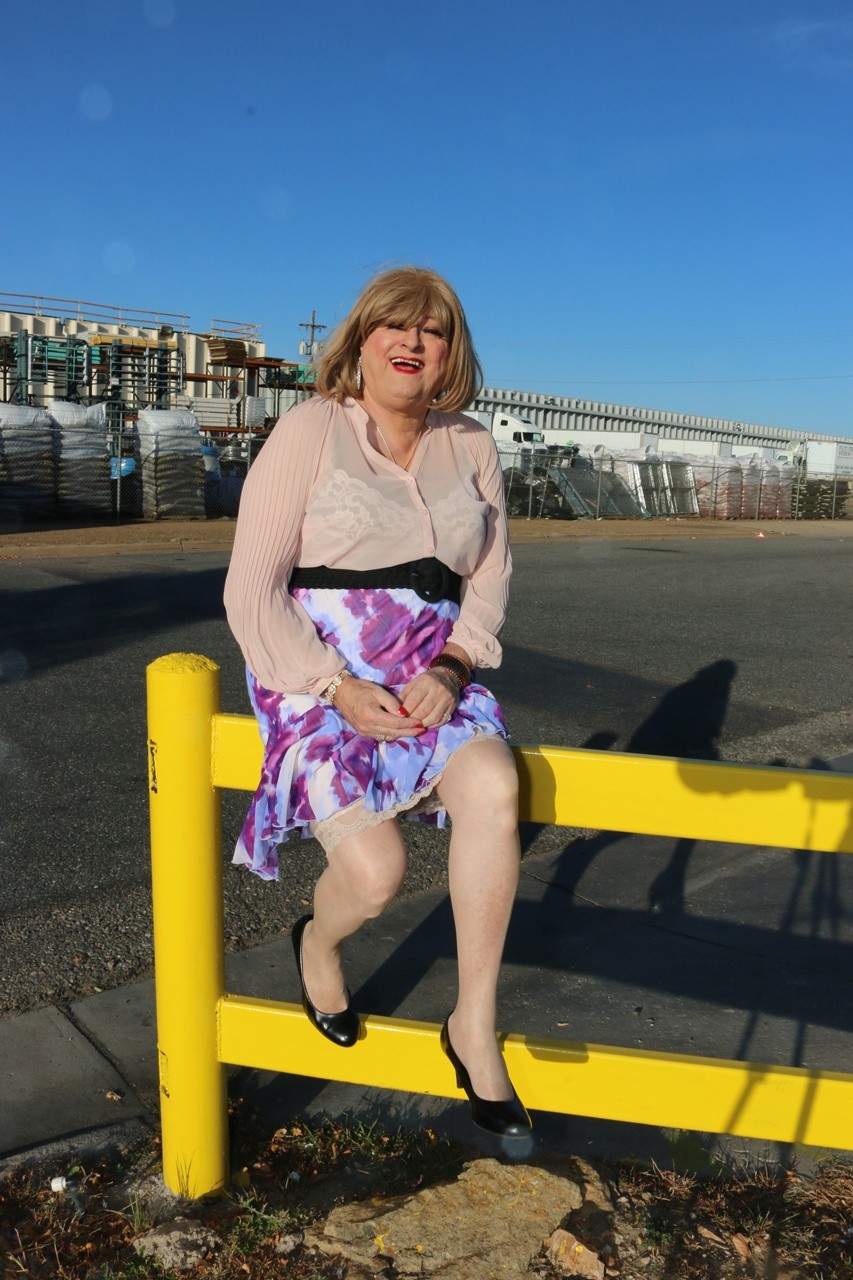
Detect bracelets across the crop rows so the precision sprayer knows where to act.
[326,669,351,707]
[429,653,472,690]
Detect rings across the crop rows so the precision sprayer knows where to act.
[375,734,385,742]
[442,713,451,722]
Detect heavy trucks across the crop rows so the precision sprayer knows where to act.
[462,409,550,461]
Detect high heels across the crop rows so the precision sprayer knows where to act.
[442,1010,531,1137]
[292,913,359,1048]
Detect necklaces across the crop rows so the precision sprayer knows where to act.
[361,397,422,469]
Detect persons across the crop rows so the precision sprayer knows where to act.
[224,268,533,1140]
